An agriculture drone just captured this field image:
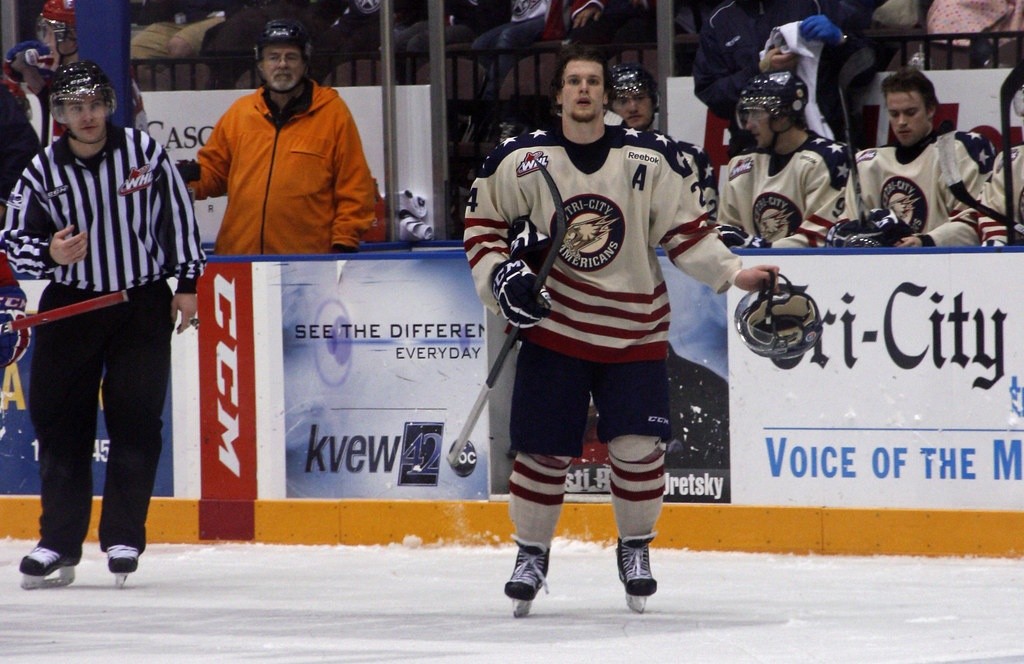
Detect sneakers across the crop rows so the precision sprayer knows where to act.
[19,538,82,589]
[106,543,138,587]
[504,535,550,617]
[615,531,658,613]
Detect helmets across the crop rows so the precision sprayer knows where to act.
[740,68,809,118]
[4,42,54,86]
[609,62,658,102]
[42,0,76,26]
[740,291,824,361]
[50,61,114,106]
[257,17,312,66]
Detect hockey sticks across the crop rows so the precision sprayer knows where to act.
[937,120,1024,236]
[0,277,179,335]
[447,161,569,465]
[837,47,876,221]
[1000,58,1024,245]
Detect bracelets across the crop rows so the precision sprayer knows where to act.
[727,270,741,286]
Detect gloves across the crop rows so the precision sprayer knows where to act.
[716,225,773,250]
[824,219,883,249]
[493,257,552,328]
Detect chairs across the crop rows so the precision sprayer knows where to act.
[126,37,976,158]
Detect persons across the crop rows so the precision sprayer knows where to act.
[0,0,1024,249]
[463,51,780,617]
[0,60,207,590]
[0,252,30,370]
